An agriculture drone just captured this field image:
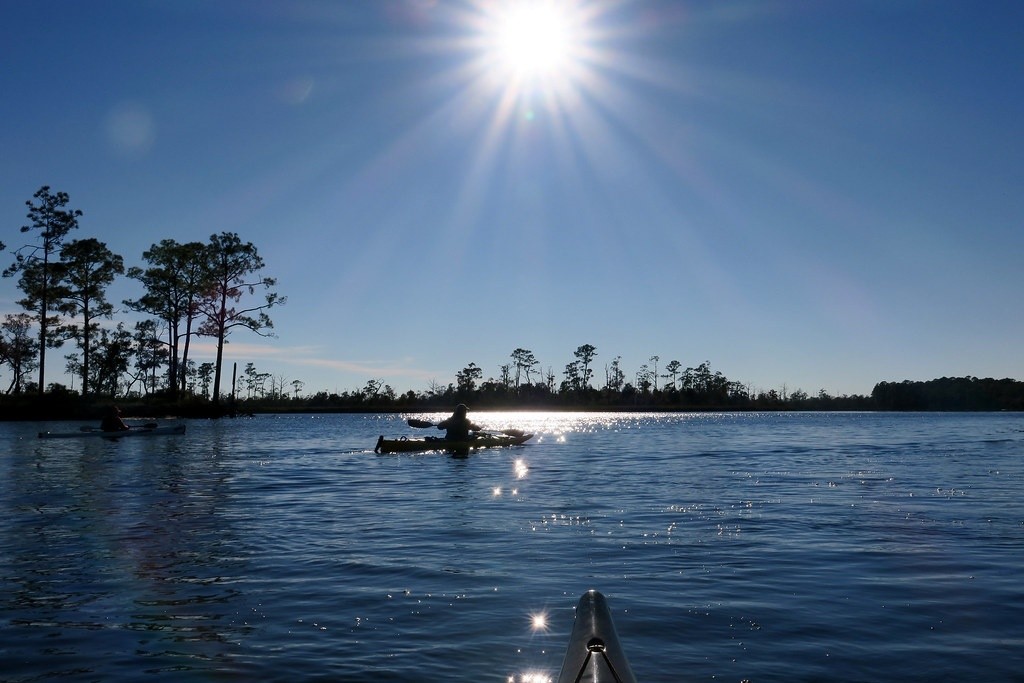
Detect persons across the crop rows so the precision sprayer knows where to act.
[101,406,128,431]
[437,404,481,441]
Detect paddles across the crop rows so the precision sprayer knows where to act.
[407,419,523,438]
[79,421,159,431]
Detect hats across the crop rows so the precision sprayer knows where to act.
[456,404,470,411]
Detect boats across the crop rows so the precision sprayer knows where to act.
[375,434,535,454]
[39,424,187,437]
